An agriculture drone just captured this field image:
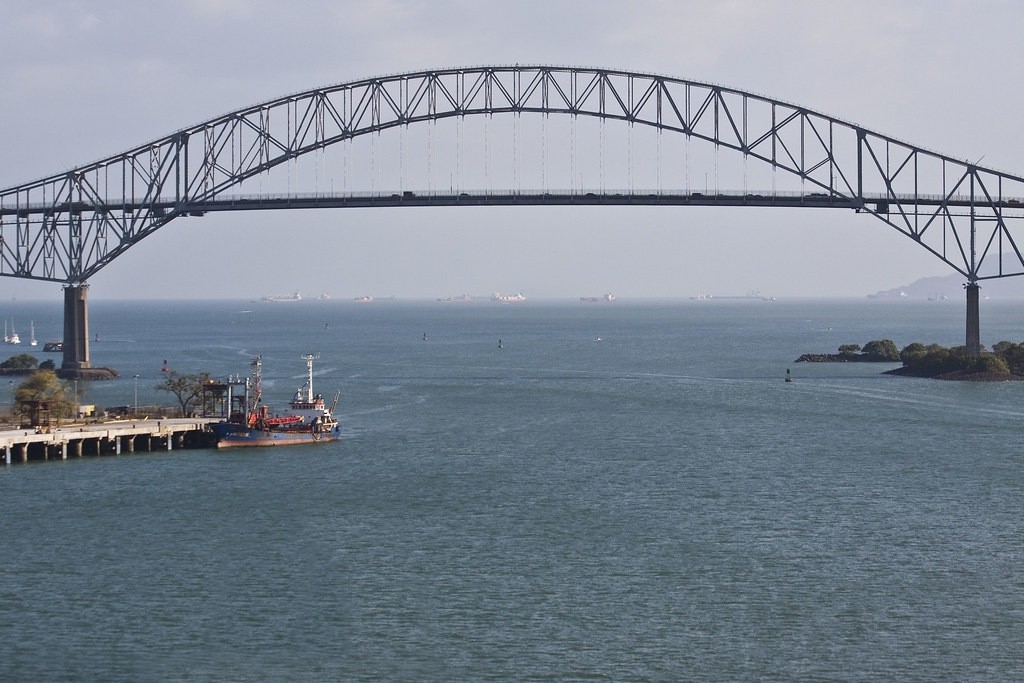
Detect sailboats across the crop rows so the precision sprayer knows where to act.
[30,319,38,346]
[3,317,21,345]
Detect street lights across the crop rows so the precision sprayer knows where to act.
[73,378,78,422]
[132,375,140,416]
[9,380,16,428]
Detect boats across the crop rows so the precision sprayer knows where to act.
[208,351,343,449]
